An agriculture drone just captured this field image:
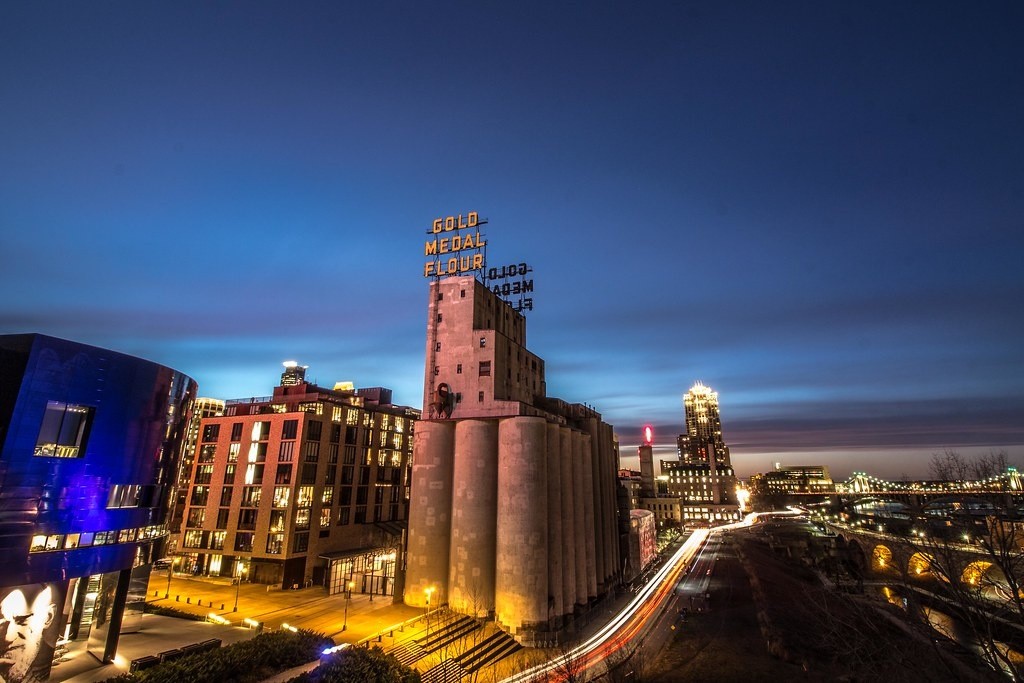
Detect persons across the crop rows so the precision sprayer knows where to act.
[0,582,57,683]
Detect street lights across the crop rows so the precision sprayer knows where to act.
[233,562,245,612]
[165,558,180,599]
[424,587,435,651]
[342,581,354,631]
